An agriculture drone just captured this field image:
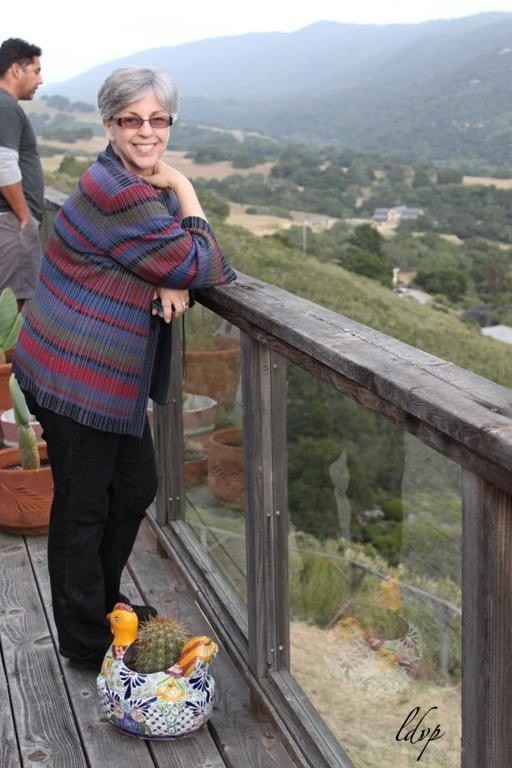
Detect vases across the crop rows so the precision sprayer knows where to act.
[208,426,247,511]
[0,407,44,447]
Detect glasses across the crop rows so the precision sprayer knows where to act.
[110,115,173,129]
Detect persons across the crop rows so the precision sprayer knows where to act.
[11,66,237,671]
[0,38,43,308]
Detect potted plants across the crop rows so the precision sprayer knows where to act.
[95,603,218,742]
[328,567,424,680]
[146,391,218,446]
[0,286,26,411]
[183,300,241,429]
[0,374,57,537]
[180,438,209,490]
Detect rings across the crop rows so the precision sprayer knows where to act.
[181,302,190,310]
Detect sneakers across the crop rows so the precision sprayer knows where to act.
[131,605,159,626]
